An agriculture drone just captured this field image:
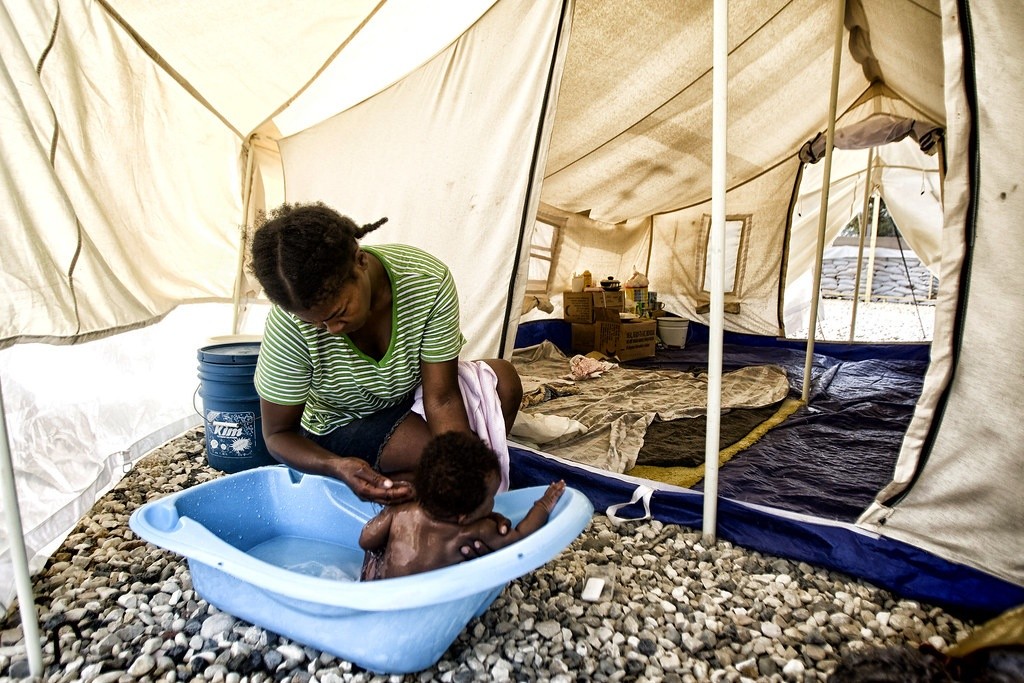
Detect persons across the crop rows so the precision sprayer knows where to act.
[252,206,524,508]
[359,431,566,581]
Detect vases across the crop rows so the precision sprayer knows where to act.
[657,315,691,349]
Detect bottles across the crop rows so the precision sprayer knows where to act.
[583,270,591,287]
[636,300,647,320]
[648,292,657,309]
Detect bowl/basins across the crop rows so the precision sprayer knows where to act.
[650,302,665,311]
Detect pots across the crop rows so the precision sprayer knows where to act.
[600,276,620,292]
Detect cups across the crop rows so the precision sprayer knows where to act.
[572,279,583,292]
[619,313,640,319]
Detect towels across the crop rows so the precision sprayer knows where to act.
[509,409,589,444]
[410,360,511,494]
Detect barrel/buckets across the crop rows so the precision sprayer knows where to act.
[197,341,280,474]
[209,334,266,343]
[657,316,689,348]
[625,287,648,317]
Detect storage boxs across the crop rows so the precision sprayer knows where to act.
[625,286,648,314]
[560,289,625,324]
[594,316,656,363]
[649,291,658,307]
[571,322,596,350]
